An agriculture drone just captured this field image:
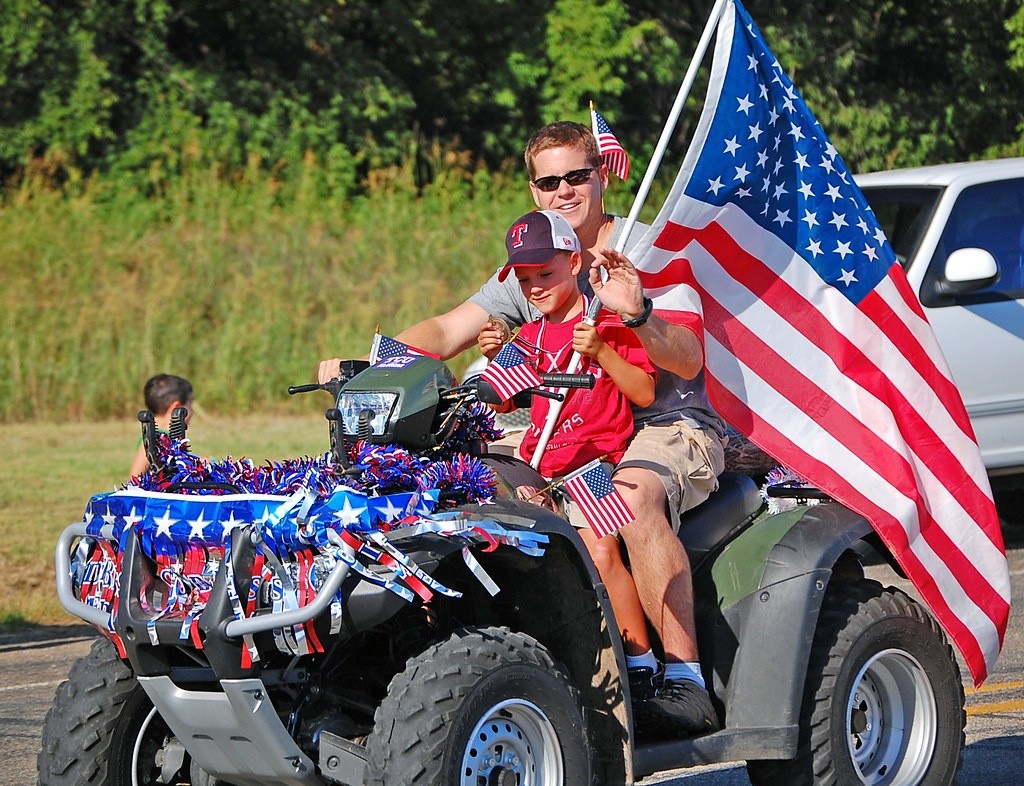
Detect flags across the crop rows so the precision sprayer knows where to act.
[596,0,1011,689]
[369,324,440,366]
[590,100,629,182]
[479,341,544,402]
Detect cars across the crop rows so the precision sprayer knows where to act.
[839,155,1024,543]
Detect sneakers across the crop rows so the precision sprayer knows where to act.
[635,678,721,736]
[626,657,665,712]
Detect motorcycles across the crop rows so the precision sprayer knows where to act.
[30,346,970,786]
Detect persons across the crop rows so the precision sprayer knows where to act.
[129,374,192,480]
[317,117,727,738]
[478,210,665,709]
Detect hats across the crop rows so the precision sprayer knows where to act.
[498,210,582,282]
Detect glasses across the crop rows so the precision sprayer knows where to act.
[533,166,599,192]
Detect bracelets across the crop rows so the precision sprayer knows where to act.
[620,297,654,327]
